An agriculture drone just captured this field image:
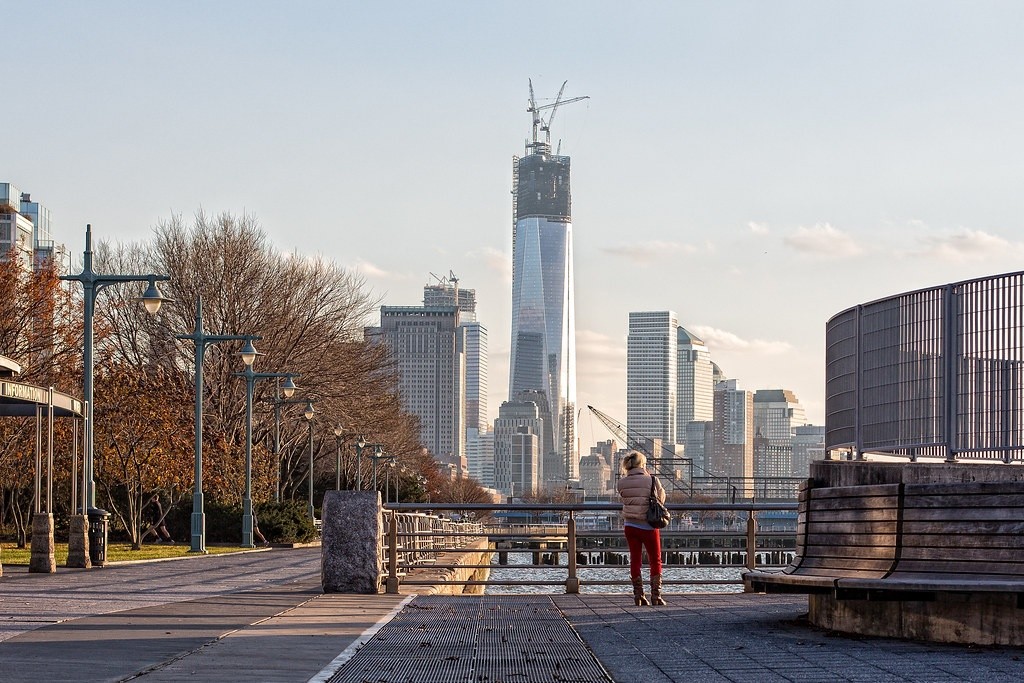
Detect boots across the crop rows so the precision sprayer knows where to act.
[649,573,667,605]
[629,571,650,606]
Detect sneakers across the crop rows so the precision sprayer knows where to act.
[166,539,175,545]
[154,537,162,544]
[264,541,269,547]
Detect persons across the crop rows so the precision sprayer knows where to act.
[148,494,176,544]
[617,450,669,606]
[241,491,270,547]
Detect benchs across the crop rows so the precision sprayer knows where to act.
[742,482,1024,601]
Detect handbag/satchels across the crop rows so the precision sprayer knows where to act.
[646,493,671,528]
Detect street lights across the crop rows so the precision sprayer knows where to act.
[57,223,175,514]
[332,420,441,515]
[266,377,320,504]
[229,362,304,549]
[173,293,268,554]
